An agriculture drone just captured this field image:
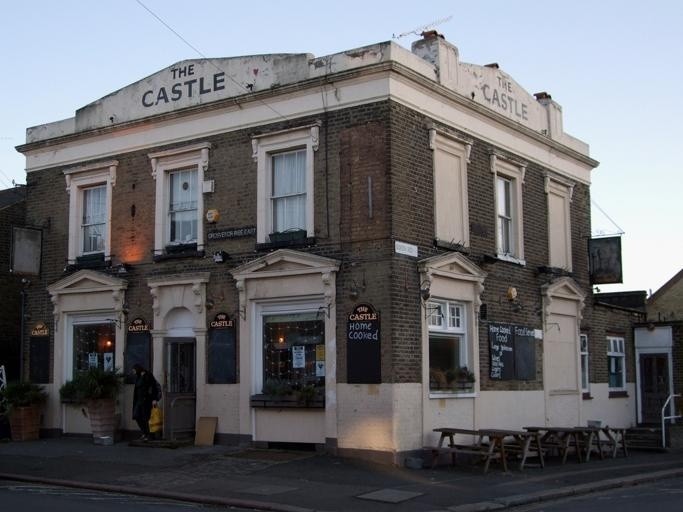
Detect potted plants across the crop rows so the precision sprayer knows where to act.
[59,364,129,447]
[0,380,50,442]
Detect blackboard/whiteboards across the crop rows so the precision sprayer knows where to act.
[27,318,51,384]
[346,302,382,384]
[488,320,536,380]
[123,317,152,384]
[205,311,237,384]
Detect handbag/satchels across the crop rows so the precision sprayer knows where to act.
[148,381,161,400]
[149,408,162,432]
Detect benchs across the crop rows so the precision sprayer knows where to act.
[422,440,630,475]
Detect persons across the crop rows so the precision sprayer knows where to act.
[132,363,162,443]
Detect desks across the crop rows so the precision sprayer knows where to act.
[431,427,629,463]
